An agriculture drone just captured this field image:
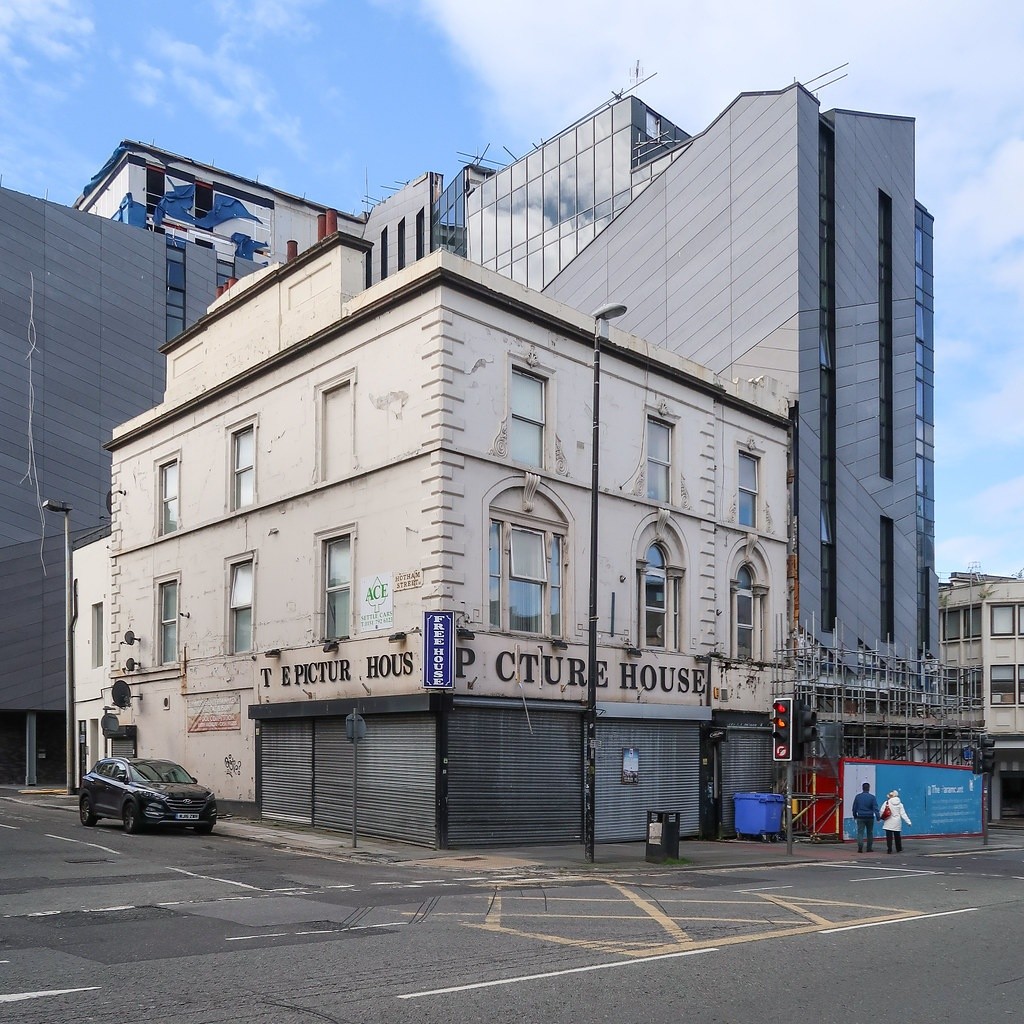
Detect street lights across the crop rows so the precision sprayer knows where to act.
[584,302,630,865]
[43,499,78,796]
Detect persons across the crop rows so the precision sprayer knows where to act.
[852,783,880,853]
[879,790,912,854]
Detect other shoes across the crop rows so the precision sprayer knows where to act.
[858,850,862,853]
[897,850,902,852]
[888,850,891,853]
[866,849,873,852]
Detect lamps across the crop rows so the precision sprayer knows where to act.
[554,638,568,650]
[627,648,642,658]
[264,649,280,660]
[390,631,407,643]
[457,630,476,641]
[323,640,339,653]
[694,655,711,664]
[752,661,766,672]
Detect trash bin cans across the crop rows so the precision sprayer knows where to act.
[645,810,680,864]
[733,793,785,843]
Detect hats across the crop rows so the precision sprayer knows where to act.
[887,790,898,799]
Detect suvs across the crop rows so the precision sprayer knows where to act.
[77,755,219,835]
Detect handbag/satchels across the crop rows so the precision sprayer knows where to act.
[881,800,891,820]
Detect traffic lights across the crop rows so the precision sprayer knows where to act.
[972,746,984,774]
[797,707,818,745]
[976,732,996,773]
[768,698,794,762]
